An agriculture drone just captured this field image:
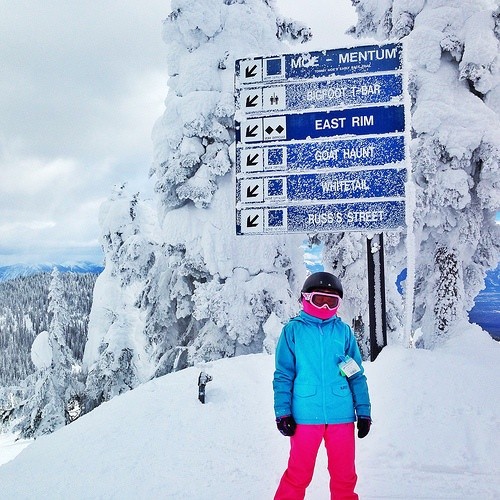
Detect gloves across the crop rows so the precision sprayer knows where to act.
[276,414,296,436]
[357,418,371,438]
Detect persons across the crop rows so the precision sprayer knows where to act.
[273,272,371,500]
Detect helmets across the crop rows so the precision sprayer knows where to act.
[301,272,343,302]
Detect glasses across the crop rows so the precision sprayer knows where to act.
[301,292,342,310]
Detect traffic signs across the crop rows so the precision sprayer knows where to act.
[235,166,413,204]
[235,71,414,117]
[231,104,413,146]
[235,141,412,174]
[234,198,408,234]
[233,41,405,85]
